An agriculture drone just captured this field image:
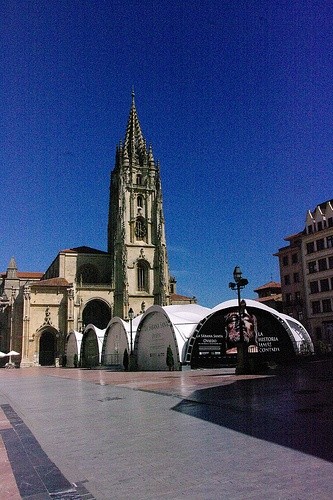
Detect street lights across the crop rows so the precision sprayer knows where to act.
[128,308,137,370]
[54,332,60,358]
[228,266,252,374]
